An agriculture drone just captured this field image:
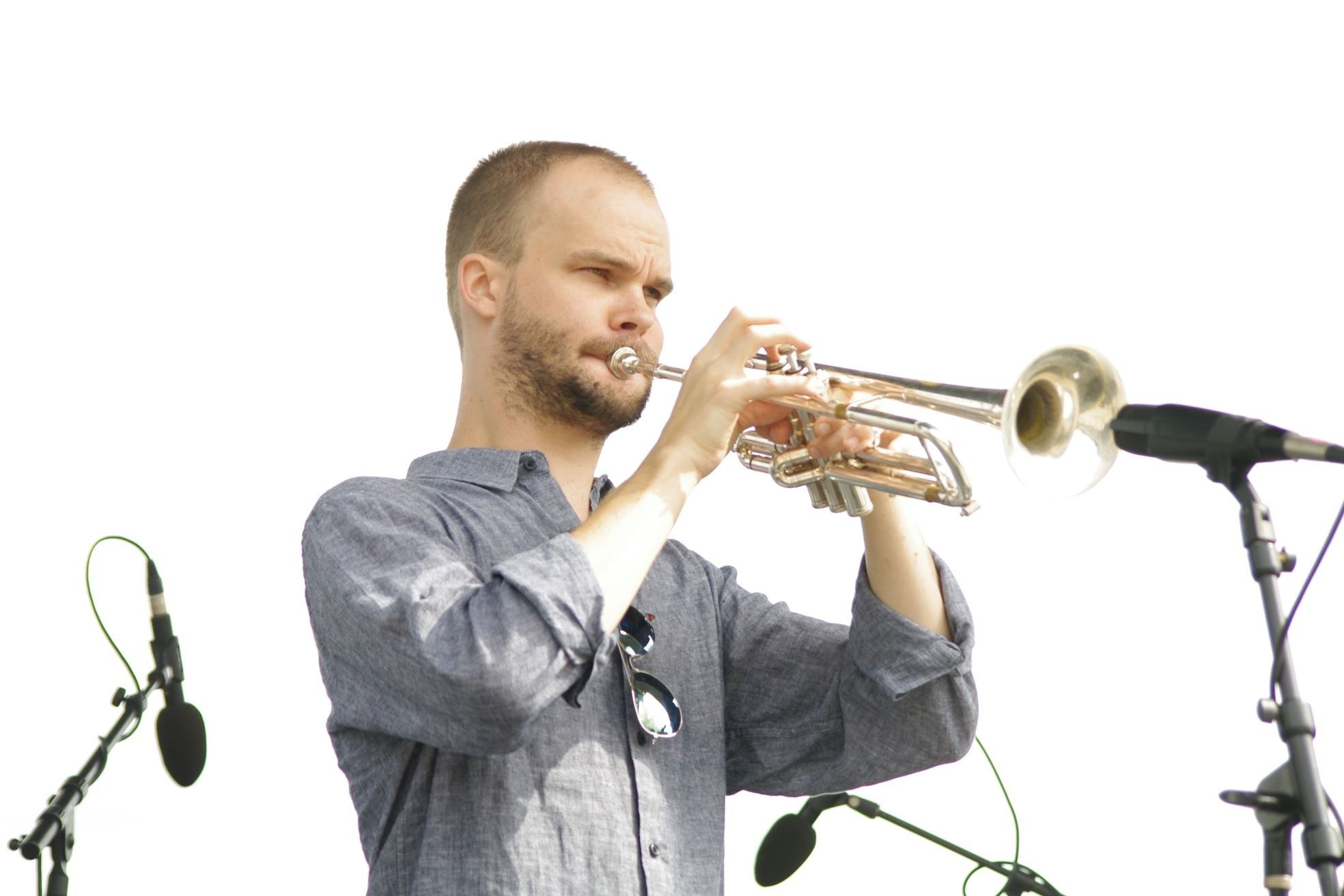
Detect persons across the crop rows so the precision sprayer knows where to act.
[302,139,976,896]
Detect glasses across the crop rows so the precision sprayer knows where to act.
[616,605,682,738]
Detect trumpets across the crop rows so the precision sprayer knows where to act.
[608,343,1130,517]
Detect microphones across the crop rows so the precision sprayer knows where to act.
[146,561,207,788]
[755,795,832,887]
[1113,404,1344,466]
